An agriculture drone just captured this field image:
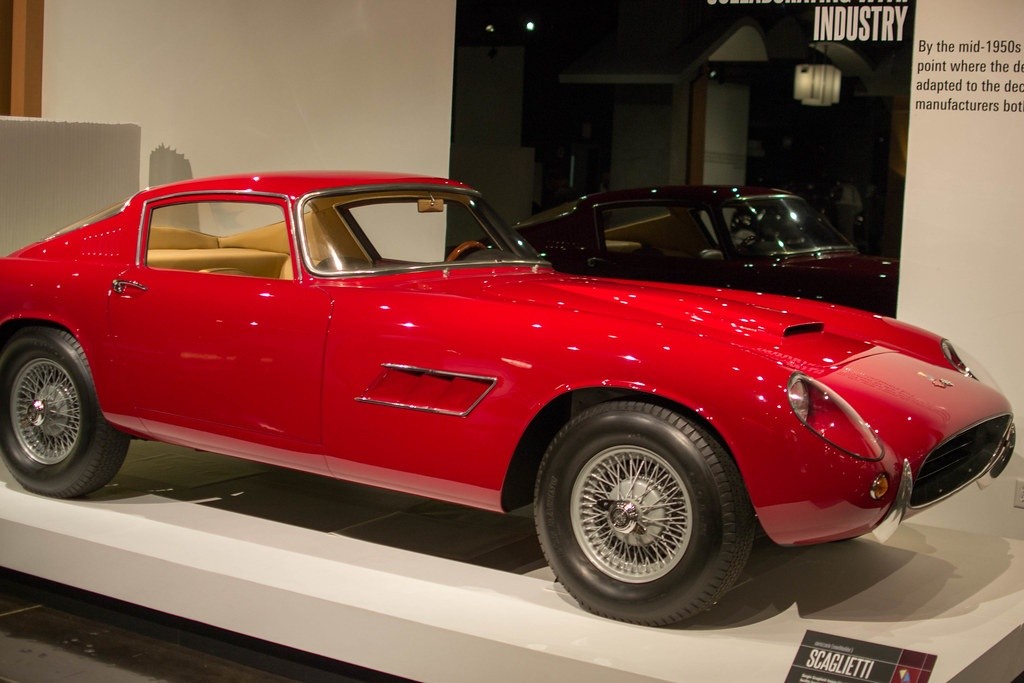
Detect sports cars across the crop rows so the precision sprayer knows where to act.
[503,183,899,319]
[0,168,1018,627]
